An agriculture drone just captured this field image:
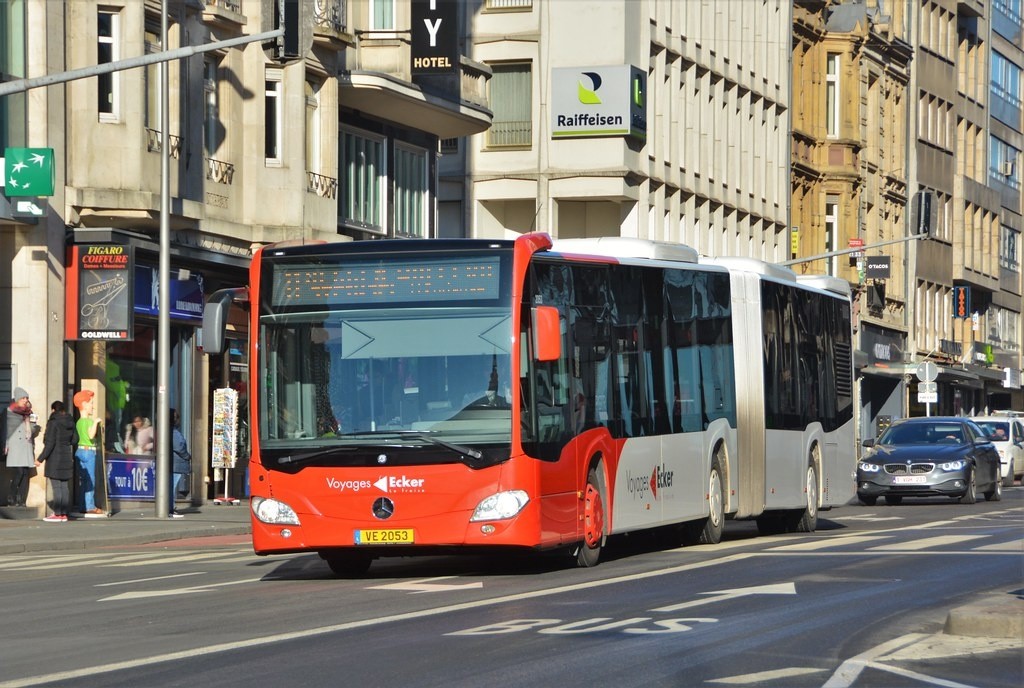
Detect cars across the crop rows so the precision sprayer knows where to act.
[970,417,1024,488]
[203,232,869,575]
[857,415,1003,506]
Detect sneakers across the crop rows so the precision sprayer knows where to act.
[62,515,68,522]
[169,507,184,518]
[43,513,62,522]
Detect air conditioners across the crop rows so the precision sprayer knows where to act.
[1005,162,1014,176]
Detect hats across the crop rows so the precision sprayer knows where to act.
[14,387,29,402]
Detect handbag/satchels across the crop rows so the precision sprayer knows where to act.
[178,472,190,497]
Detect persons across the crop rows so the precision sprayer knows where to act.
[169,408,193,518]
[945,432,962,444]
[34,400,80,523]
[124,414,154,455]
[991,425,1016,442]
[460,355,513,412]
[357,361,402,421]
[0,385,37,507]
[74,389,105,516]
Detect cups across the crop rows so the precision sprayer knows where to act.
[29,414,37,423]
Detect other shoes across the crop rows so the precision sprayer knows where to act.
[15,500,26,507]
[7,500,19,506]
